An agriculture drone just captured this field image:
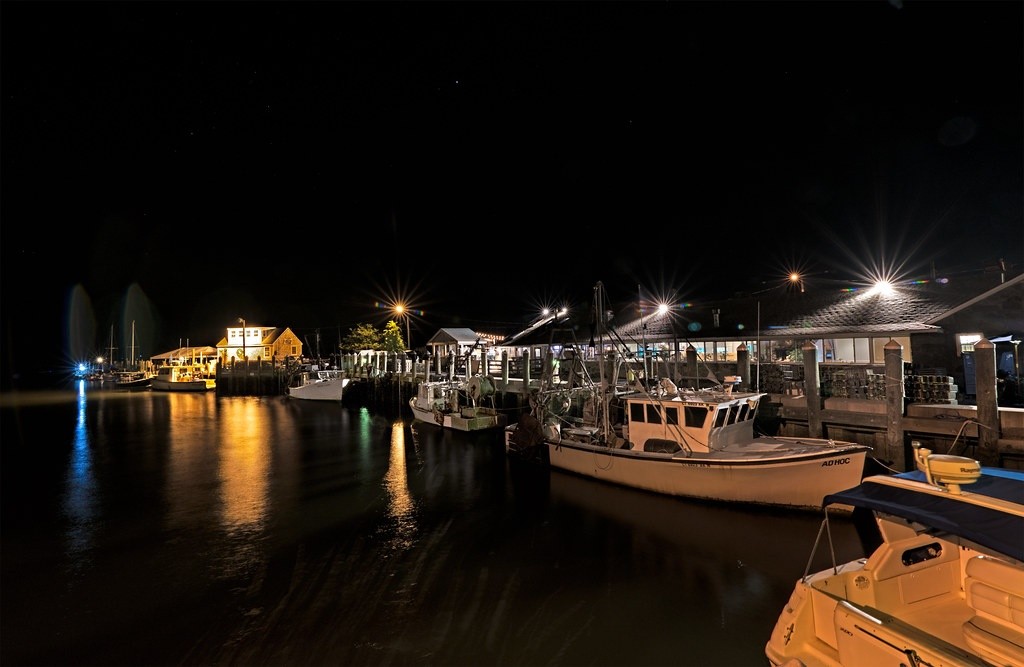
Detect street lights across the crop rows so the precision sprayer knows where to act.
[395,305,411,352]
[98,357,104,373]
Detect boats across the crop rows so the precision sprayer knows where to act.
[287,360,351,401]
[149,364,218,391]
[764,439,1024,667]
[409,373,506,432]
[505,278,878,517]
[112,369,152,390]
